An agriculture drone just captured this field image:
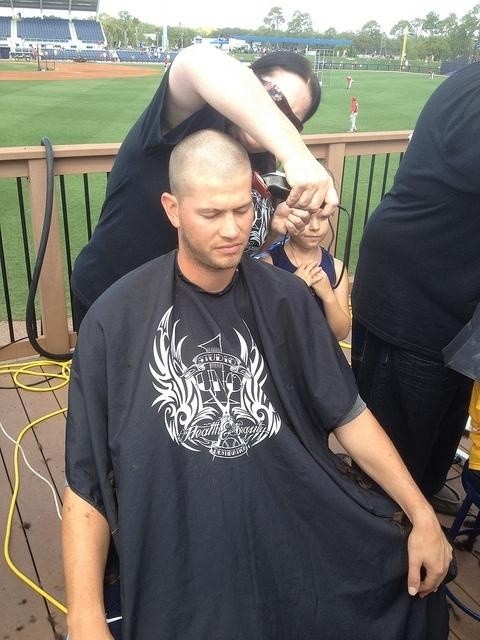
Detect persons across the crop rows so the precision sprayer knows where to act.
[56,126,453,640]
[463,380,480,495]
[69,42,342,338]
[348,98,360,131]
[256,167,351,340]
[347,75,353,90]
[164,58,168,72]
[352,60,480,503]
[427,70,436,79]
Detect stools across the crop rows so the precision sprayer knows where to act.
[449,459,480,548]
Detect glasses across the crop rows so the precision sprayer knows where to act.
[257,77,303,133]
